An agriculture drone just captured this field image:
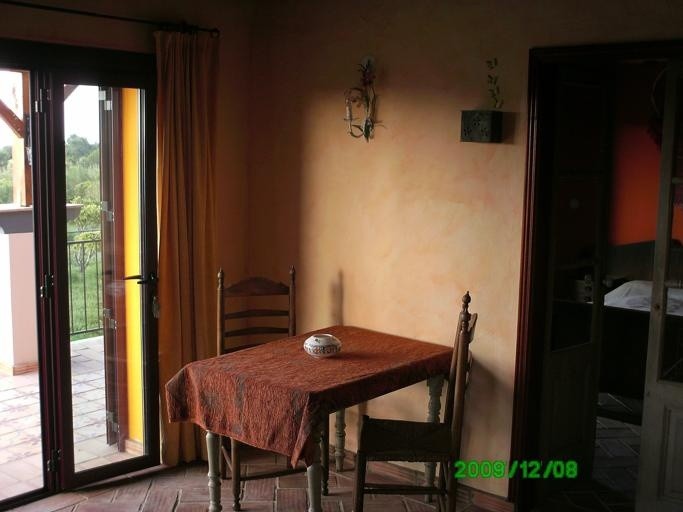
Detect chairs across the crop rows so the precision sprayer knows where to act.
[355,291,477,512]
[216,264,298,512]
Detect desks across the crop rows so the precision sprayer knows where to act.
[165,325,454,512]
[585,279,683,396]
